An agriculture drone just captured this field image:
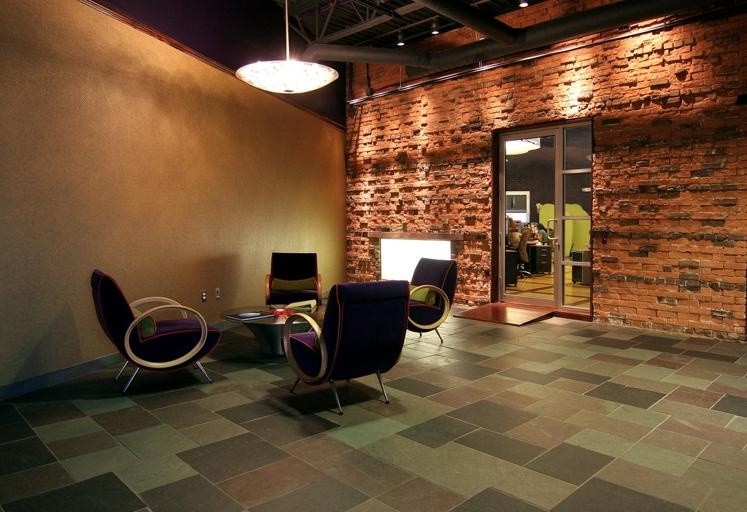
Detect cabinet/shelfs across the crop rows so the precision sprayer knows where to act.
[571,251,593,284]
[527,244,551,274]
[505,249,518,287]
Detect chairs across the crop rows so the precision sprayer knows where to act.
[262,249,321,304]
[282,276,411,416]
[88,265,221,394]
[406,254,460,341]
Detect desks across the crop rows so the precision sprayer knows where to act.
[218,303,327,357]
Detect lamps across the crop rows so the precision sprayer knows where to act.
[235,2,339,97]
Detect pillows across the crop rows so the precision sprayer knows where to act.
[311,326,324,350]
[128,305,158,339]
[408,285,437,306]
[272,275,315,290]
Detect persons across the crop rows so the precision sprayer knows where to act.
[512,223,547,246]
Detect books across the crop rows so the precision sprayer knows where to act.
[224,309,276,322]
